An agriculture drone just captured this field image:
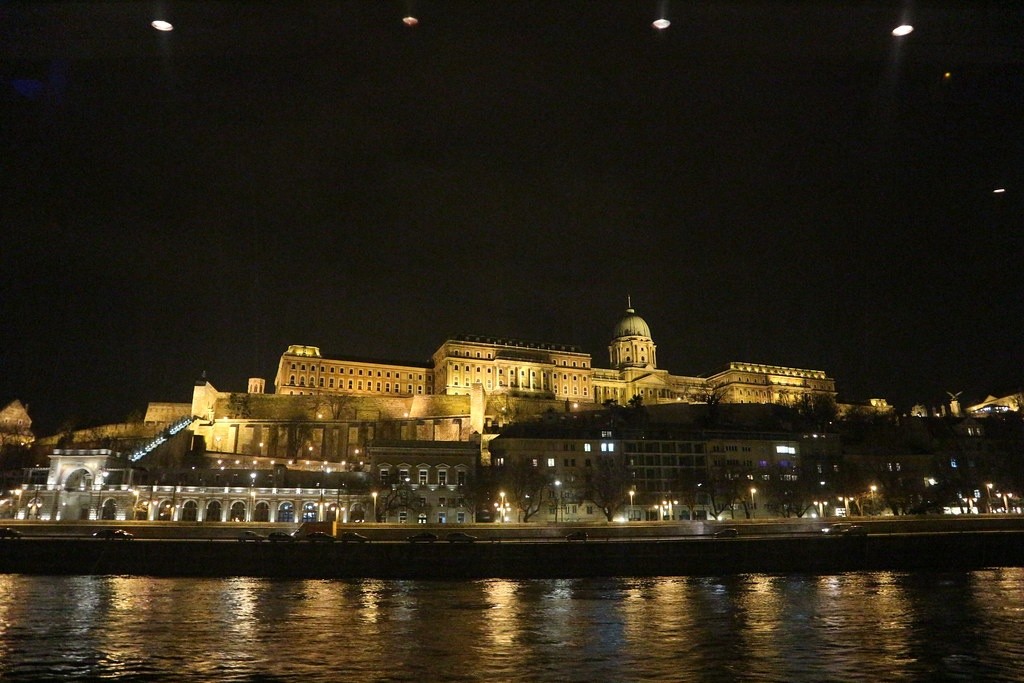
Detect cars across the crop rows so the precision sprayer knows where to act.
[848,525,868,537]
[242,530,264,541]
[444,531,478,544]
[342,532,367,543]
[714,529,738,537]
[565,530,589,541]
[407,533,439,542]
[822,523,852,535]
[92,530,134,539]
[306,532,338,543]
[266,531,295,543]
[0,527,24,539]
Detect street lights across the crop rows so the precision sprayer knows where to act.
[870,486,876,508]
[751,488,755,518]
[499,492,505,523]
[629,490,634,521]
[372,492,378,517]
[555,481,563,522]
